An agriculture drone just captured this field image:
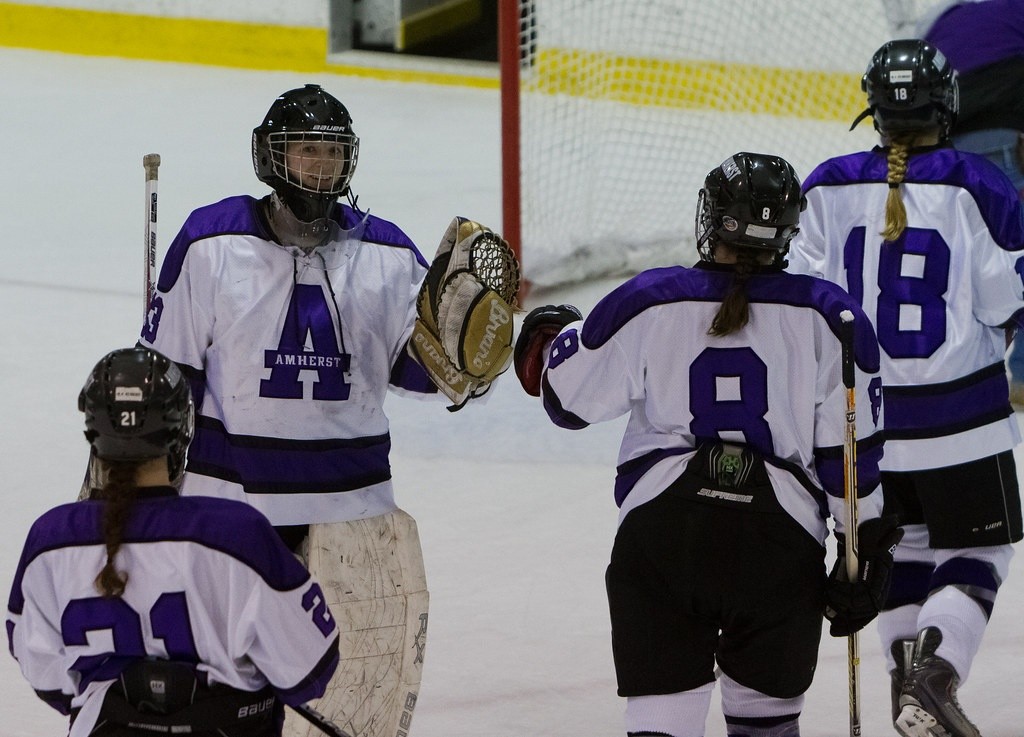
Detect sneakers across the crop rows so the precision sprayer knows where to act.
[889,626,983,737]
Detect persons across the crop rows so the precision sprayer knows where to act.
[920,0,1023,410]
[516,151,905,737]
[782,39,1024,735]
[5,348,341,737]
[136,85,528,737]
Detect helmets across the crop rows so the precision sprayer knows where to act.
[251,83,361,203]
[76,348,195,488]
[849,38,961,144]
[695,151,808,272]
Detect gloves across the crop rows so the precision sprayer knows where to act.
[514,304,584,398]
[824,516,905,637]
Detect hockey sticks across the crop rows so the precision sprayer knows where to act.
[142,153,163,323]
[840,308,863,737]
[288,700,352,737]
